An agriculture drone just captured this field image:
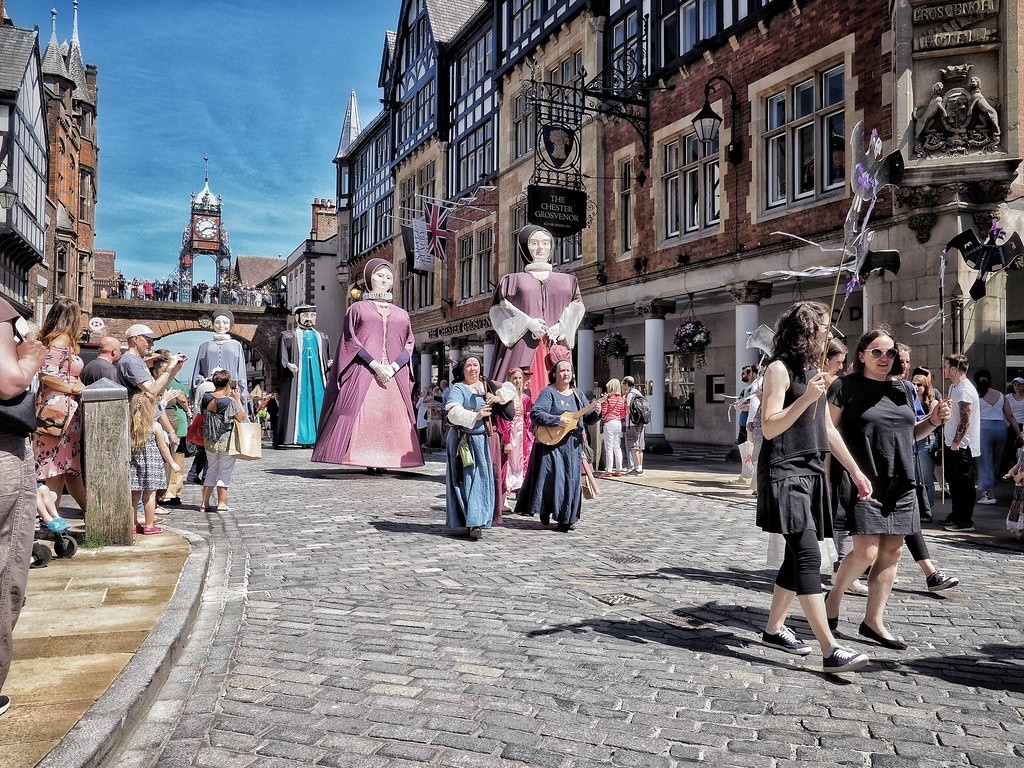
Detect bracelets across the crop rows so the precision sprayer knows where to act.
[927,417,939,428]
[164,369,172,374]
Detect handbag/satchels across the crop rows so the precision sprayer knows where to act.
[0,328,37,439]
[35,405,67,438]
[186,413,204,447]
[229,413,262,461]
[930,449,942,466]
[600,421,603,433]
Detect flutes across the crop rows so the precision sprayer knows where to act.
[484,381,493,436]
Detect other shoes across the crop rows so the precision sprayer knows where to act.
[54,517,71,530]
[470,526,481,540]
[600,472,611,477]
[40,520,63,532]
[540,513,550,526]
[729,477,750,485]
[0,695,10,715]
[559,523,575,530]
[132,478,232,535]
[762,490,998,671]
[613,472,621,477]
[627,470,643,476]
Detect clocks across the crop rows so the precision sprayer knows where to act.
[195,217,218,239]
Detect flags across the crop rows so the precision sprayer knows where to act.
[401,203,448,274]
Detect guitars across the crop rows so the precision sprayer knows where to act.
[537,391,614,446]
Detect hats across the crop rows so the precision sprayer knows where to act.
[124,324,161,341]
[1011,377,1024,384]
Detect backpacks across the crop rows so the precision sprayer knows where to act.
[626,392,651,424]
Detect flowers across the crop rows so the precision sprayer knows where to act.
[671,320,713,373]
[596,333,629,367]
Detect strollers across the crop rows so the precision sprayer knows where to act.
[28,452,79,569]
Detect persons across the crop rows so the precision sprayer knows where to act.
[0,296,45,716]
[585,375,645,477]
[130,392,181,535]
[77,335,123,386]
[310,257,426,476]
[32,296,86,515]
[731,300,1024,673]
[187,366,246,513]
[412,353,603,539]
[143,347,192,514]
[247,391,280,444]
[34,483,71,532]
[412,379,450,448]
[117,323,189,524]
[269,302,333,451]
[191,307,247,411]
[486,222,586,405]
[116,272,285,307]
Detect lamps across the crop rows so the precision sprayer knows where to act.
[691,77,742,161]
[0,162,20,210]
[351,271,365,298]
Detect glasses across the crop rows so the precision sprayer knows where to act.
[742,372,746,375]
[863,349,898,359]
[914,383,923,387]
[820,324,832,331]
